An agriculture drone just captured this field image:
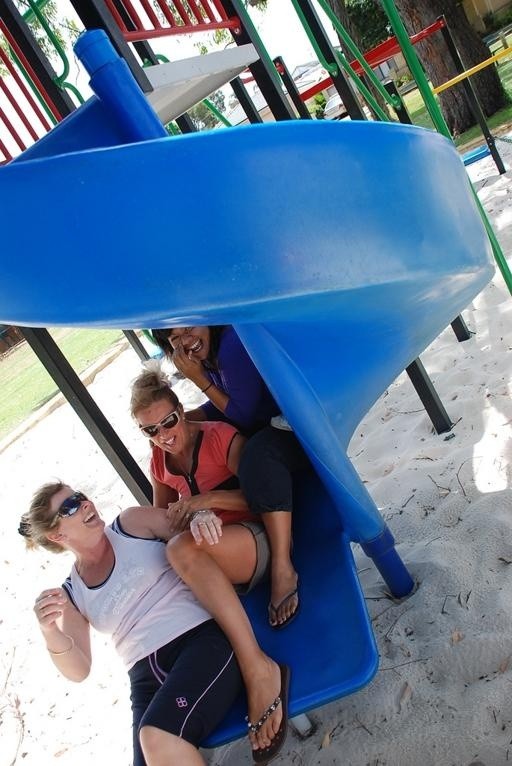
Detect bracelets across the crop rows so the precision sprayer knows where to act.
[192,509,213,519]
[201,382,214,393]
[46,634,75,656]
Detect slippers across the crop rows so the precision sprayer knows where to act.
[266,574,303,631]
[245,664,291,764]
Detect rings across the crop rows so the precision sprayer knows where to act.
[185,513,191,518]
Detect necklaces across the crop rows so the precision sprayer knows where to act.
[177,462,194,484]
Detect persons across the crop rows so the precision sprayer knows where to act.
[131,365,294,764]
[152,324,313,630]
[19,483,245,766]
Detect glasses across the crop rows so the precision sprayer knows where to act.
[137,407,181,440]
[49,491,89,527]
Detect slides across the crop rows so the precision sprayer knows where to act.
[0,95,496,749]
